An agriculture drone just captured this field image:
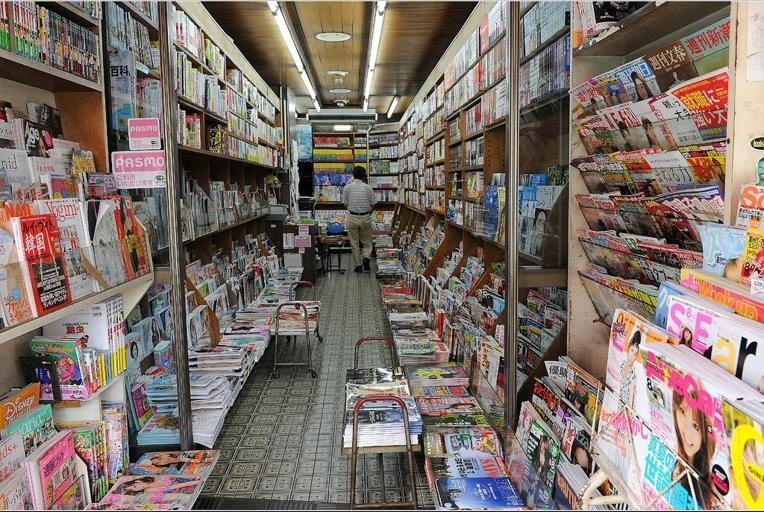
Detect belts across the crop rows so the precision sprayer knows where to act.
[349,211,370,216]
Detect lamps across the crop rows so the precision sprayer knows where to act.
[317,33,351,42]
[327,71,349,77]
[268,2,320,111]
[333,79,344,85]
[335,99,349,108]
[362,2,387,110]
[328,89,351,94]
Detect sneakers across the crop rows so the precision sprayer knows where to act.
[363,260,370,270]
[354,266,363,273]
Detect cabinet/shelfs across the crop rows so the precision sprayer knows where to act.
[374,1,762,511]
[0,0,286,510]
[269,111,383,252]
